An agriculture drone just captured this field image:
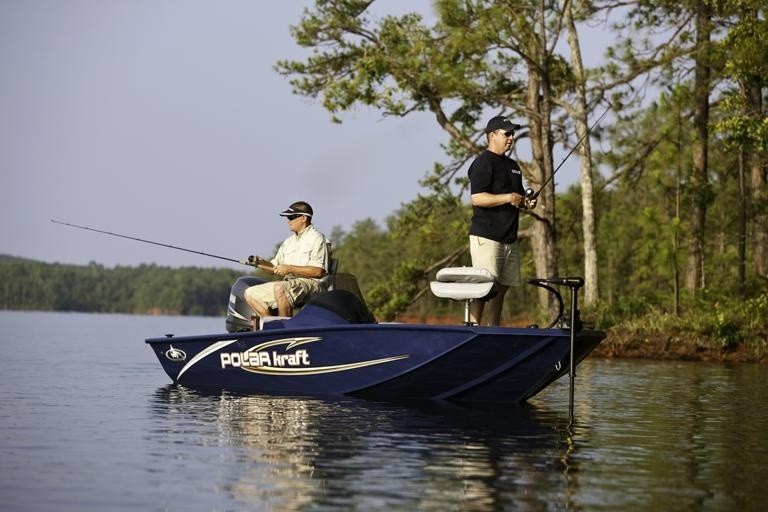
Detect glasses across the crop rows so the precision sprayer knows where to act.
[504,131,514,137]
[286,214,303,220]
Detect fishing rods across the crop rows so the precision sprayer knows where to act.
[524,98,620,202]
[51,220,276,271]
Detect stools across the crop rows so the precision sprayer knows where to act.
[427,265,504,326]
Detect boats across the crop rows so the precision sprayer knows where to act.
[144,319,609,406]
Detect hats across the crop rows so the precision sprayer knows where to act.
[280,202,313,219]
[486,116,521,133]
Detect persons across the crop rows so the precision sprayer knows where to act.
[466,116,539,327]
[242,200,335,320]
